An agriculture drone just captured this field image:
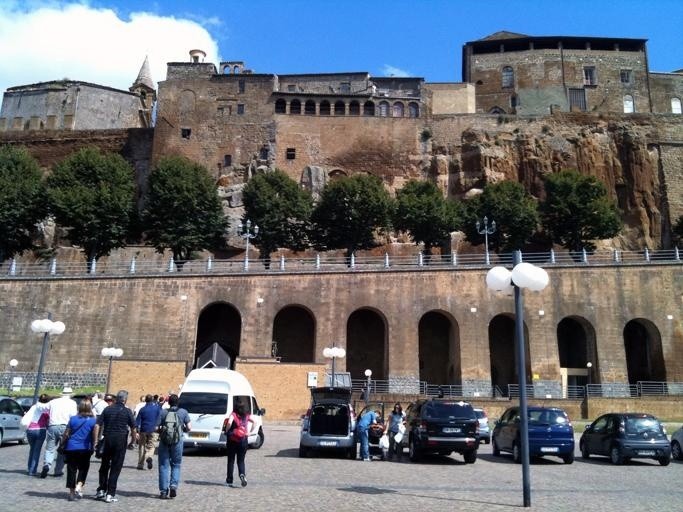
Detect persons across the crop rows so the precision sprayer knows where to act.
[379,428,390,460]
[19,387,192,502]
[384,402,408,462]
[356,410,381,460]
[222,400,249,487]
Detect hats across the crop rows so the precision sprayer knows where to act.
[60,387,73,396]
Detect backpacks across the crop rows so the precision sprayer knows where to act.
[159,407,182,445]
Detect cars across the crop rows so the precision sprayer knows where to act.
[70,394,93,411]
[577,412,671,467]
[471,407,490,446]
[671,424,683,461]
[0,396,28,447]
[15,397,40,413]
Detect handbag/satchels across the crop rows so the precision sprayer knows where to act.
[56,417,88,455]
[94,405,124,459]
[222,418,245,442]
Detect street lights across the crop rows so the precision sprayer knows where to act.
[586,361,593,395]
[236,218,259,271]
[475,216,497,263]
[484,252,549,508]
[28,311,65,407]
[6,358,18,395]
[100,341,123,397]
[363,368,372,412]
[322,341,345,387]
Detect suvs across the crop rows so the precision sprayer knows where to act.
[399,399,479,465]
[298,386,383,458]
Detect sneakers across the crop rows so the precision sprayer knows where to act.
[52,472,64,478]
[239,473,247,487]
[169,487,177,498]
[225,482,234,488]
[39,464,50,480]
[146,457,152,470]
[92,489,105,500]
[136,466,143,470]
[105,494,118,503]
[68,484,83,502]
[159,490,169,500]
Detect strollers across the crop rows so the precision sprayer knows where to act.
[367,424,386,461]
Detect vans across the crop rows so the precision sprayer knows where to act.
[174,369,263,454]
[490,406,575,465]
